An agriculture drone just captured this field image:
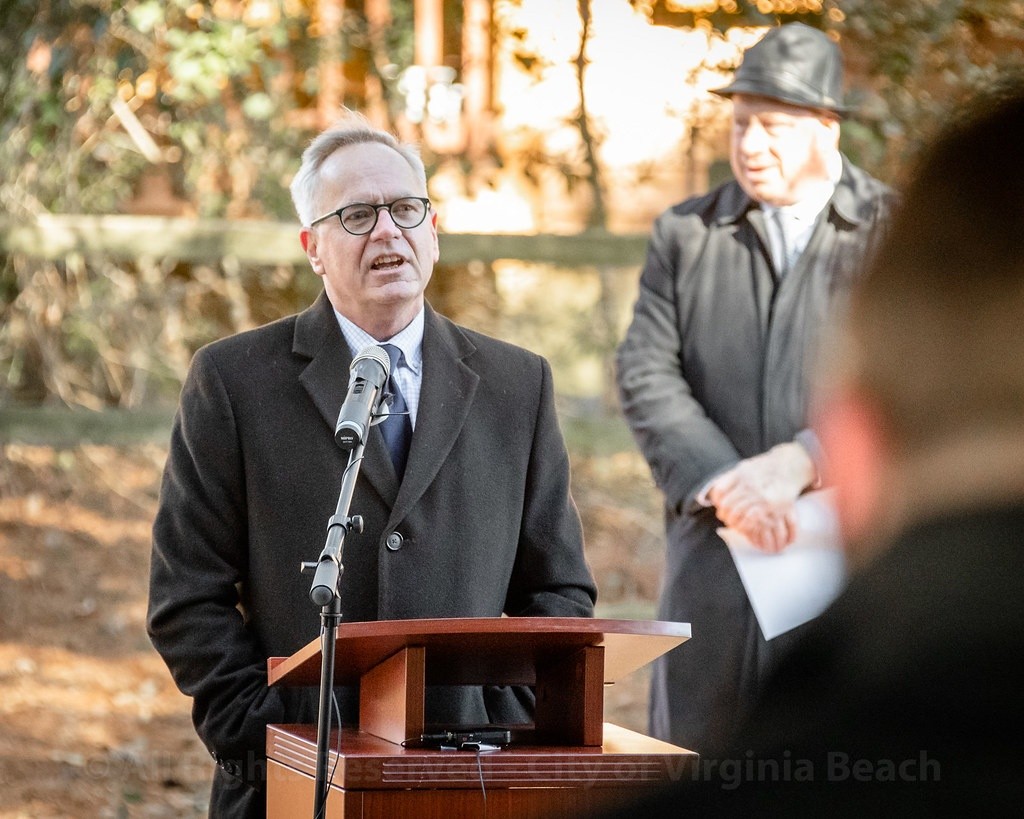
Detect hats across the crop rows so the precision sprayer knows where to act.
[707,22,860,126]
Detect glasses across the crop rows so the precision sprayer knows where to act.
[310,196,432,236]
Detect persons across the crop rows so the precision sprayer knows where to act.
[611,19,902,755]
[599,66,1024,818]
[141,120,599,819]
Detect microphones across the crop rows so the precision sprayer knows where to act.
[334,345,390,451]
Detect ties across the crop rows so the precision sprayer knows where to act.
[770,209,797,278]
[376,343,414,488]
